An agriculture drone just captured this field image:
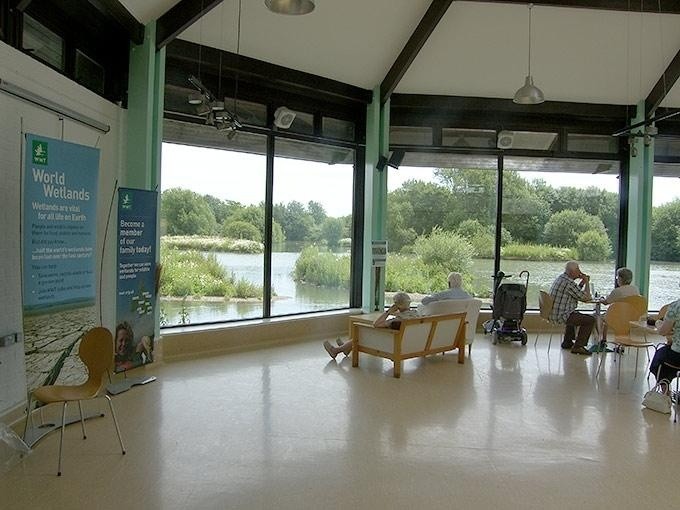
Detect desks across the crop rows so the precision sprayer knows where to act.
[629,319,659,334]
[578,293,614,352]
[347,312,397,341]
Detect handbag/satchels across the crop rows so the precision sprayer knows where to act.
[642,379,672,413]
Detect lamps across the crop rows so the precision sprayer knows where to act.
[197,103,211,117]
[189,2,203,104]
[496,130,514,149]
[215,111,228,121]
[644,123,651,147]
[216,118,245,142]
[629,132,639,158]
[272,104,296,130]
[211,2,225,111]
[510,2,546,105]
[265,1,315,16]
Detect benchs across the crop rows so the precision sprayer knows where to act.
[351,310,470,379]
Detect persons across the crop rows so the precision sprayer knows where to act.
[549,261,594,356]
[114,322,145,371]
[421,272,472,306]
[651,283,680,404]
[599,267,641,354]
[323,291,418,360]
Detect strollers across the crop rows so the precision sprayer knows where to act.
[483,267,530,347]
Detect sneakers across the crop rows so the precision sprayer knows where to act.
[600,340,607,348]
[614,347,624,353]
[561,340,592,355]
[323,341,337,358]
[336,338,349,355]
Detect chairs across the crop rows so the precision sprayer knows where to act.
[596,301,658,389]
[533,290,578,354]
[23,323,125,477]
[613,296,650,365]
[417,298,484,358]
[647,303,672,381]
[653,361,679,423]
